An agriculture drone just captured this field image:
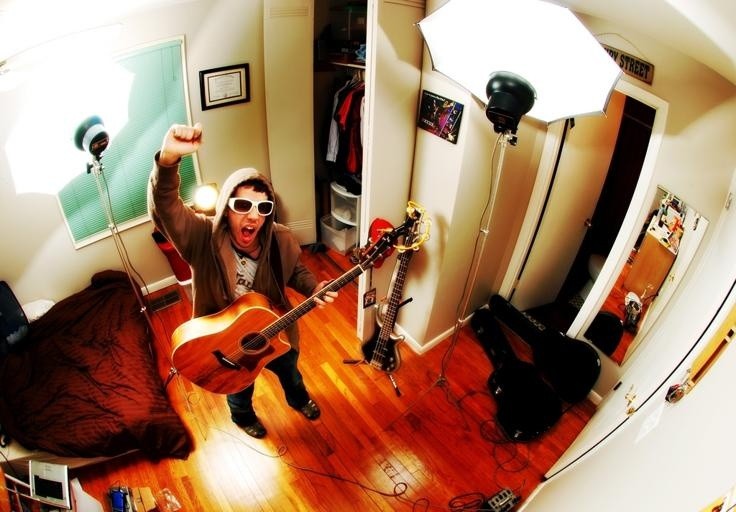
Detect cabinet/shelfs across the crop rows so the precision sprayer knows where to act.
[260,0,427,345]
[620,213,680,307]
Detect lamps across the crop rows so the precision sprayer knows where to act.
[74,115,178,397]
[387,0,627,435]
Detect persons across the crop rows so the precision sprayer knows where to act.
[146,122,339,438]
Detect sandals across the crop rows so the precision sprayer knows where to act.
[300,399,320,420]
[243,421,266,438]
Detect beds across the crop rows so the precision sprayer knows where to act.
[0,270,194,480]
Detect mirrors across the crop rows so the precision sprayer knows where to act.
[582,183,711,369]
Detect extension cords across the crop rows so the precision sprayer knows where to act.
[482,489,513,509]
[496,496,519,512]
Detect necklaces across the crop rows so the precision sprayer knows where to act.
[229,234,260,258]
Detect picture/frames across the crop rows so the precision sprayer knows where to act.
[196,60,253,112]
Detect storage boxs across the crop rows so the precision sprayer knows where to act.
[318,215,359,257]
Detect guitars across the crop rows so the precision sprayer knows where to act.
[171,230,397,394]
[362,209,430,373]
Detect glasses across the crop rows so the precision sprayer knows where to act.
[227,197,274,217]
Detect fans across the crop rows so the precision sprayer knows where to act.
[329,181,362,229]
